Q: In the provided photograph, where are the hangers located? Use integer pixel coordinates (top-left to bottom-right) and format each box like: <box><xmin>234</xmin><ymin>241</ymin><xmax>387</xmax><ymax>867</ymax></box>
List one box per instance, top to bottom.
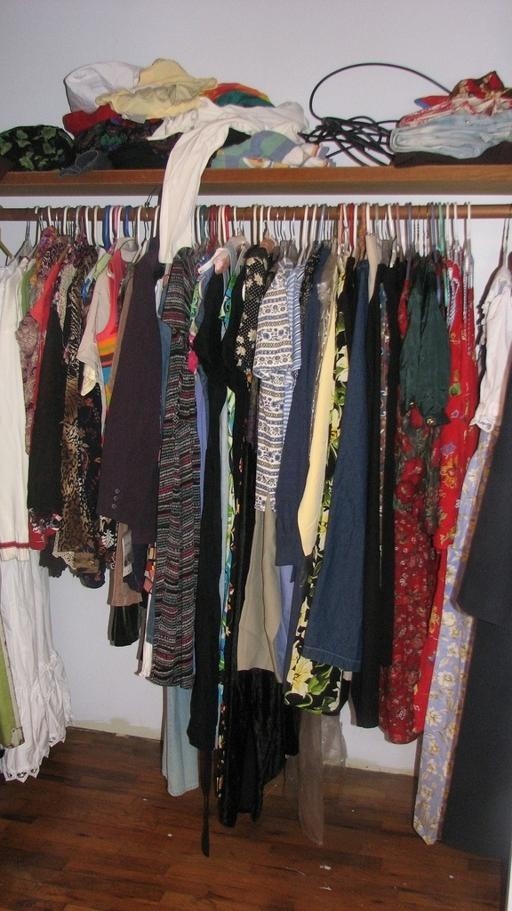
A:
<box><xmin>3</xmin><ymin>203</ymin><xmax>476</xmax><ymax>276</ymax></box>
<box><xmin>497</xmin><ymin>203</ymin><xmax>512</xmax><ymax>257</ymax></box>
